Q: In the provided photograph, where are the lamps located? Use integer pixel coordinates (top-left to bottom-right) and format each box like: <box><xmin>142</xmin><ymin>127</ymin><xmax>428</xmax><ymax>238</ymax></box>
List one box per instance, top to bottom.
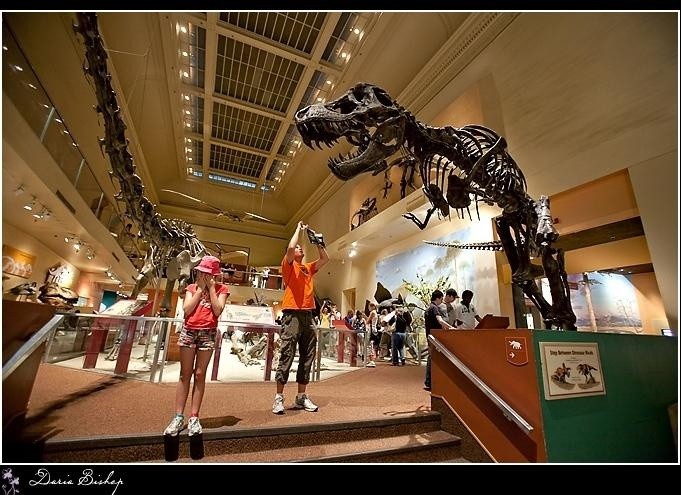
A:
<box><xmin>23</xmin><ymin>200</ymin><xmax>37</xmax><ymax>212</ymax></box>
<box><xmin>32</xmin><ymin>207</ymin><xmax>46</xmax><ymax>220</ymax></box>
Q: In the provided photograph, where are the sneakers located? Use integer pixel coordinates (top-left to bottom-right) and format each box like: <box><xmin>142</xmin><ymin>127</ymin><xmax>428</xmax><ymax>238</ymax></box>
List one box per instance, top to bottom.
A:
<box><xmin>366</xmin><ymin>362</ymin><xmax>376</xmax><ymax>368</ymax></box>
<box><xmin>294</xmin><ymin>395</ymin><xmax>319</xmax><ymax>412</ymax></box>
<box><xmin>271</xmin><ymin>397</ymin><xmax>285</xmax><ymax>414</ymax></box>
<box><xmin>163</xmin><ymin>416</ymin><xmax>186</xmax><ymax>437</ymax></box>
<box><xmin>187</xmin><ymin>416</ymin><xmax>203</xmax><ymax>437</ymax></box>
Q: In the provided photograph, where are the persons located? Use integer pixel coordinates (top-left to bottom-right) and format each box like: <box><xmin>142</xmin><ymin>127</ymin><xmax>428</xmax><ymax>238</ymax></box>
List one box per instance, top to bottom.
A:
<box><xmin>271</xmin><ymin>220</ymin><xmax>330</xmax><ymax>415</ymax></box>
<box><xmin>162</xmin><ymin>256</ymin><xmax>230</xmax><ymax>438</ymax></box>
<box><xmin>438</xmin><ymin>288</ymin><xmax>457</xmax><ymax>331</ymax></box>
<box><xmin>453</xmin><ymin>289</ymin><xmax>483</xmax><ymax>331</ymax></box>
<box><xmin>423</xmin><ymin>290</ymin><xmax>456</xmax><ymax>390</ymax></box>
<box><xmin>319</xmin><ymin>300</ymin><xmax>331</xmax><ymax>328</ymax></box>
<box><xmin>328</xmin><ymin>303</ymin><xmax>341</xmax><ymax>326</ymax></box>
<box><xmin>365</xmin><ymin>303</ymin><xmax>379</xmax><ymax>367</ymax></box>
<box><xmin>390</xmin><ymin>307</ymin><xmax>395</xmax><ymax>312</ymax></box>
<box><xmin>392</xmin><ymin>308</ymin><xmax>406</xmax><ymax>366</ymax></box>
<box><xmin>375</xmin><ymin>309</ymin><xmax>391</xmax><ymax>362</ymax></box>
<box><xmin>377</xmin><ymin>309</ymin><xmax>386</xmax><ymax>327</ymax></box>
<box><xmin>312</xmin><ymin>310</ymin><xmax>319</xmax><ymax>323</ymax></box>
<box><xmin>344</xmin><ymin>310</ymin><xmax>353</xmax><ymax>327</ymax></box>
<box><xmin>352</xmin><ymin>310</ymin><xmax>365</xmax><ymax>358</ymax></box>
<box><xmin>403</xmin><ymin>311</ymin><xmax>419</xmax><ymax>361</ymax></box>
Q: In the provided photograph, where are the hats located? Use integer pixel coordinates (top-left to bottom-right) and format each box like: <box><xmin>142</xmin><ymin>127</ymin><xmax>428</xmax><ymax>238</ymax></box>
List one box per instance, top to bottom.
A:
<box><xmin>194</xmin><ymin>254</ymin><xmax>225</xmax><ymax>278</ymax></box>
<box><xmin>447</xmin><ymin>288</ymin><xmax>460</xmax><ymax>298</ymax></box>
